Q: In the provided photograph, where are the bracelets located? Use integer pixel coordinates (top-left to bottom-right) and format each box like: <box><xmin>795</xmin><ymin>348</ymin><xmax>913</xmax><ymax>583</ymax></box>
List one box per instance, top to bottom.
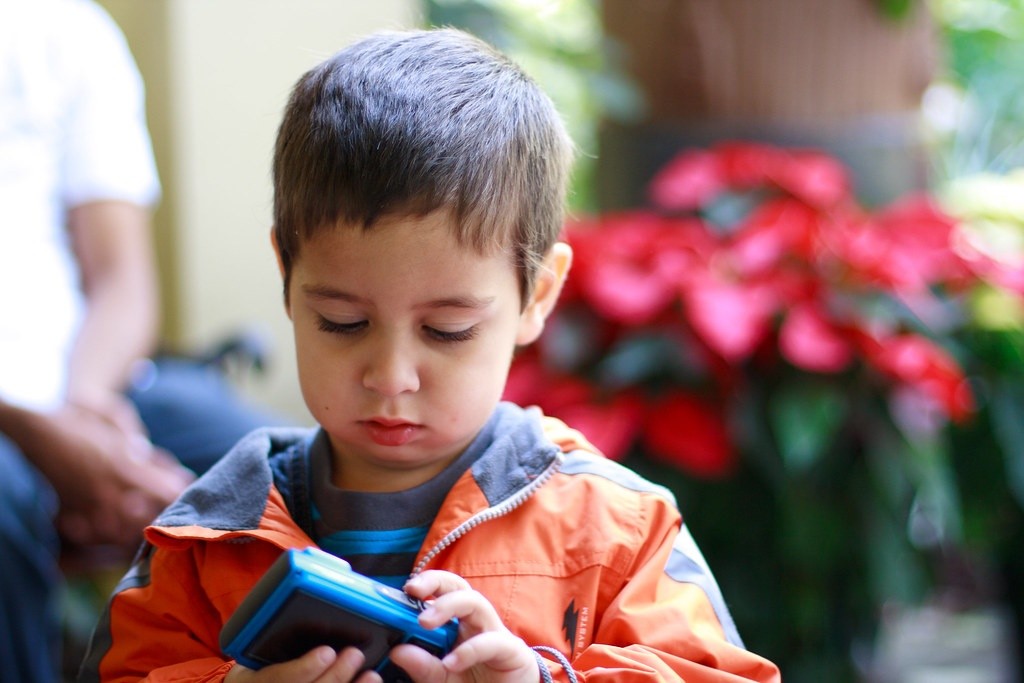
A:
<box><xmin>531</xmin><ymin>645</ymin><xmax>577</xmax><ymax>683</ymax></box>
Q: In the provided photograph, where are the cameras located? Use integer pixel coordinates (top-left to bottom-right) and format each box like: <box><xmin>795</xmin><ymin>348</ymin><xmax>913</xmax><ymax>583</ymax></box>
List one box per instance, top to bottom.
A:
<box><xmin>219</xmin><ymin>547</ymin><xmax>459</xmax><ymax>683</ymax></box>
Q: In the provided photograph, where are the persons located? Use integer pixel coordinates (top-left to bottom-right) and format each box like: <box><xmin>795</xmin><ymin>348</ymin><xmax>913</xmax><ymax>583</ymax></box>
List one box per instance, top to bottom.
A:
<box><xmin>0</xmin><ymin>0</ymin><xmax>276</xmax><ymax>683</ymax></box>
<box><xmin>77</xmin><ymin>24</ymin><xmax>782</xmax><ymax>682</ymax></box>
